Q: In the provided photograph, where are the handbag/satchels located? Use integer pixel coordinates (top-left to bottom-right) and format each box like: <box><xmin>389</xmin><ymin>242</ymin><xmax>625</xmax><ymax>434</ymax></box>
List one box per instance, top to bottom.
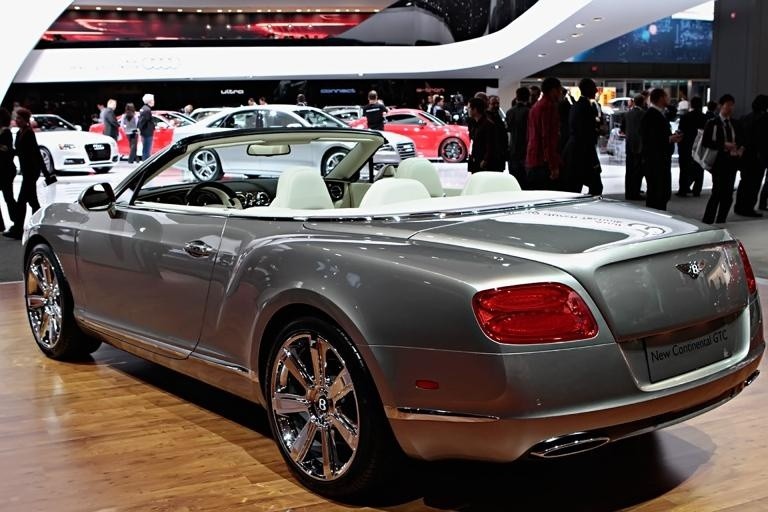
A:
<box><xmin>692</xmin><ymin>129</ymin><xmax>718</xmax><ymax>170</ymax></box>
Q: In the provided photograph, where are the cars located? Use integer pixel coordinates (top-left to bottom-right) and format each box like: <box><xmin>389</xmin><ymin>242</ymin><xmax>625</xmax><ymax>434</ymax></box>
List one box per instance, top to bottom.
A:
<box><xmin>39</xmin><ymin>0</ymin><xmax>397</xmax><ymax>44</ymax></box>
<box><xmin>601</xmin><ymin>97</ymin><xmax>634</xmax><ymax>120</ymax></box>
<box><xmin>169</xmin><ymin>103</ymin><xmax>417</xmax><ymax>179</ymax></box>
<box><xmin>349</xmin><ymin>108</ymin><xmax>472</xmax><ymax>164</ymax></box>
<box><xmin>88</xmin><ymin>111</ymin><xmax>198</xmax><ymax>162</ymax></box>
<box><xmin>9</xmin><ymin>113</ymin><xmax>120</xmax><ymax>177</ymax></box>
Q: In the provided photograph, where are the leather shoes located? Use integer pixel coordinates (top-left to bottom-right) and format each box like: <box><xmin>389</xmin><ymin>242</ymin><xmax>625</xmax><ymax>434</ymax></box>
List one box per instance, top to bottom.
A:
<box><xmin>1</xmin><ymin>228</ymin><xmax>22</xmax><ymax>239</ymax></box>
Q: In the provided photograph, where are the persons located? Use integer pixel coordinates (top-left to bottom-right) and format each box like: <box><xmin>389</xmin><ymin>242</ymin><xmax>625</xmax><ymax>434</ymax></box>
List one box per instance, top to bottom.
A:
<box><xmin>468</xmin><ymin>77</ymin><xmax>610</xmax><ymax>195</ymax></box>
<box><xmin>619</xmin><ymin>86</ymin><xmax>768</xmax><ymax>225</ymax></box>
<box><xmin>363</xmin><ymin>89</ymin><xmax>386</xmax><ymax>130</ymax></box>
<box><xmin>136</xmin><ymin>93</ymin><xmax>155</xmax><ymax>162</ymax></box>
<box><xmin>296</xmin><ymin>94</ymin><xmax>308</xmax><ymax>106</ymax></box>
<box><xmin>419</xmin><ymin>94</ymin><xmax>470</xmax><ymax>126</ymax></box>
<box><xmin>258</xmin><ymin>97</ymin><xmax>268</xmax><ymax>106</ymax></box>
<box><xmin>248</xmin><ymin>98</ymin><xmax>257</xmax><ymax>106</ymax></box>
<box><xmin>102</xmin><ymin>99</ymin><xmax>121</xmax><ymax>143</ymax></box>
<box><xmin>121</xmin><ymin>102</ymin><xmax>139</xmax><ymax>163</ymax></box>
<box><xmin>90</xmin><ymin>104</ymin><xmax>106</xmax><ymax>123</ymax></box>
<box><xmin>183</xmin><ymin>104</ymin><xmax>193</xmax><ymax>116</ymax></box>
<box><xmin>0</xmin><ymin>105</ymin><xmax>17</xmax><ymax>235</ymax></box>
<box><xmin>3</xmin><ymin>107</ymin><xmax>54</xmax><ymax>240</ymax></box>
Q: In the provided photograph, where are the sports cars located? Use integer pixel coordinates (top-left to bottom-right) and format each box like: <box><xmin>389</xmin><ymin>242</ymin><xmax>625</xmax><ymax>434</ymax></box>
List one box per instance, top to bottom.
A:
<box><xmin>20</xmin><ymin>127</ymin><xmax>766</xmax><ymax>498</ymax></box>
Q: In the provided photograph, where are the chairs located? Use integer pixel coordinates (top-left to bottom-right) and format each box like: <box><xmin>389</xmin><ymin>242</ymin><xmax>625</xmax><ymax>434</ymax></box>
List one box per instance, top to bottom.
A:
<box><xmin>392</xmin><ymin>157</ymin><xmax>444</xmax><ymax>197</ymax></box>
<box><xmin>268</xmin><ymin>168</ymin><xmax>335</xmax><ymax>209</ymax></box>
<box><xmin>462</xmin><ymin>171</ymin><xmax>522</xmax><ymax>197</ymax></box>
<box><xmin>356</xmin><ymin>177</ymin><xmax>432</xmax><ymax>209</ymax></box>
